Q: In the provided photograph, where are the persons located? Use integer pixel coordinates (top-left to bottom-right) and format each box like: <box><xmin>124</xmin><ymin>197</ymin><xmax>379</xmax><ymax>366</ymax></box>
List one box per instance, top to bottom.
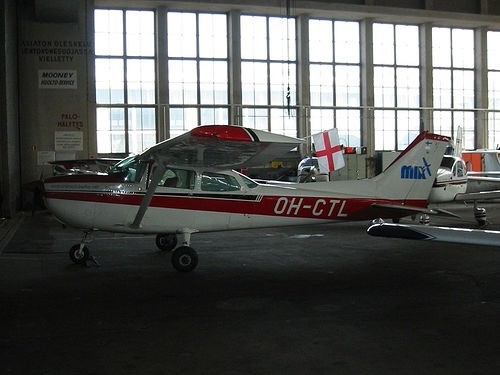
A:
<box><xmin>465</xmin><ymin>160</ymin><xmax>472</xmax><ymax>172</ymax></box>
<box><xmin>299</xmin><ymin>167</ymin><xmax>318</xmax><ymax>184</ymax></box>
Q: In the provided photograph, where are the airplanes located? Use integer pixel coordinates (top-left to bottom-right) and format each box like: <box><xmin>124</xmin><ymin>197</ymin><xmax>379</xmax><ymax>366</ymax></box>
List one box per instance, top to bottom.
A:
<box><xmin>24</xmin><ymin>124</ymin><xmax>500</xmax><ymax>272</ymax></box>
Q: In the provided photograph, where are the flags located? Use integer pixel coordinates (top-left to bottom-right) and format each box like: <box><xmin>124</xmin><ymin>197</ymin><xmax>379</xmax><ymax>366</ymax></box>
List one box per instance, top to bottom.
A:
<box><xmin>311</xmin><ymin>127</ymin><xmax>345</xmax><ymax>174</ymax></box>
